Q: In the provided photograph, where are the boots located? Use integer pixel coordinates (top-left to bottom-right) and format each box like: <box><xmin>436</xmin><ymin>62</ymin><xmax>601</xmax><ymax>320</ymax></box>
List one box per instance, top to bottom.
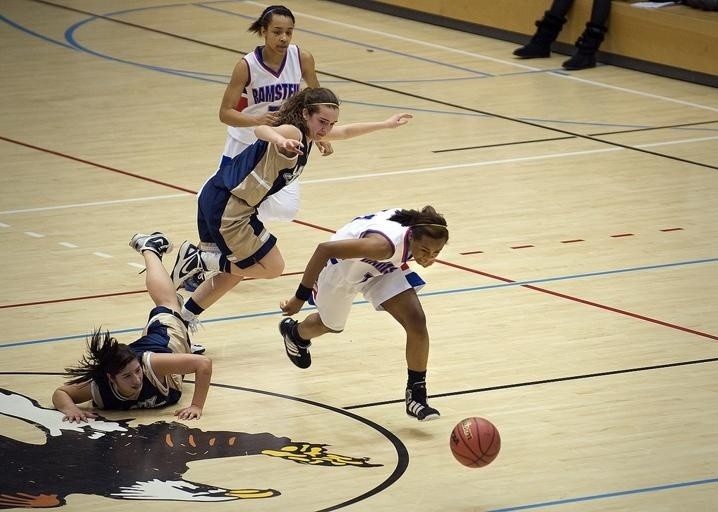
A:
<box><xmin>511</xmin><ymin>9</ymin><xmax>569</xmax><ymax>60</ymax></box>
<box><xmin>561</xmin><ymin>22</ymin><xmax>608</xmax><ymax>71</ymax></box>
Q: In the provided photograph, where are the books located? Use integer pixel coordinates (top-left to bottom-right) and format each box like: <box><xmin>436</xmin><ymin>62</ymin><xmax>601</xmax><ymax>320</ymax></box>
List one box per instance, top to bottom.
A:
<box><xmin>629</xmin><ymin>2</ymin><xmax>676</xmax><ymax>10</ymax></box>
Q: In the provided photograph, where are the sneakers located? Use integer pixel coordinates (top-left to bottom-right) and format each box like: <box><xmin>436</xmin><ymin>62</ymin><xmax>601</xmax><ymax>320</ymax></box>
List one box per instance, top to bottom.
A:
<box><xmin>128</xmin><ymin>230</ymin><xmax>175</xmax><ymax>259</ymax></box>
<box><xmin>168</xmin><ymin>241</ymin><xmax>204</xmax><ymax>311</ymax></box>
<box><xmin>278</xmin><ymin>317</ymin><xmax>313</xmax><ymax>369</ymax></box>
<box><xmin>188</xmin><ymin>341</ymin><xmax>207</xmax><ymax>356</ymax></box>
<box><xmin>403</xmin><ymin>379</ymin><xmax>441</xmax><ymax>422</ymax></box>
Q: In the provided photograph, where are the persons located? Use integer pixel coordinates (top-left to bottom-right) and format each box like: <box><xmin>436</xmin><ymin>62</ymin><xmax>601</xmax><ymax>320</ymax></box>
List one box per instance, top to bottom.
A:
<box><xmin>511</xmin><ymin>0</ymin><xmax>614</xmax><ymax>71</ymax></box>
<box><xmin>182</xmin><ymin>7</ymin><xmax>333</xmax><ymax>295</ymax></box>
<box><xmin>170</xmin><ymin>88</ymin><xmax>413</xmax><ymax>353</ymax></box>
<box><xmin>277</xmin><ymin>205</ymin><xmax>450</xmax><ymax>420</ymax></box>
<box><xmin>52</xmin><ymin>232</ymin><xmax>214</xmax><ymax>425</ymax></box>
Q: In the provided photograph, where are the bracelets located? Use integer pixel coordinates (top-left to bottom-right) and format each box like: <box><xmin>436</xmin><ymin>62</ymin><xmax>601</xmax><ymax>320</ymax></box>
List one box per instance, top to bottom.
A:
<box><xmin>295</xmin><ymin>283</ymin><xmax>312</xmax><ymax>301</ymax></box>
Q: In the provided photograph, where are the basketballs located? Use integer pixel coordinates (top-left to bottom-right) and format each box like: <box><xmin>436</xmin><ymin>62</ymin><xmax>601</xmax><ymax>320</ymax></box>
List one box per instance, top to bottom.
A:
<box><xmin>450</xmin><ymin>417</ymin><xmax>500</xmax><ymax>467</ymax></box>
